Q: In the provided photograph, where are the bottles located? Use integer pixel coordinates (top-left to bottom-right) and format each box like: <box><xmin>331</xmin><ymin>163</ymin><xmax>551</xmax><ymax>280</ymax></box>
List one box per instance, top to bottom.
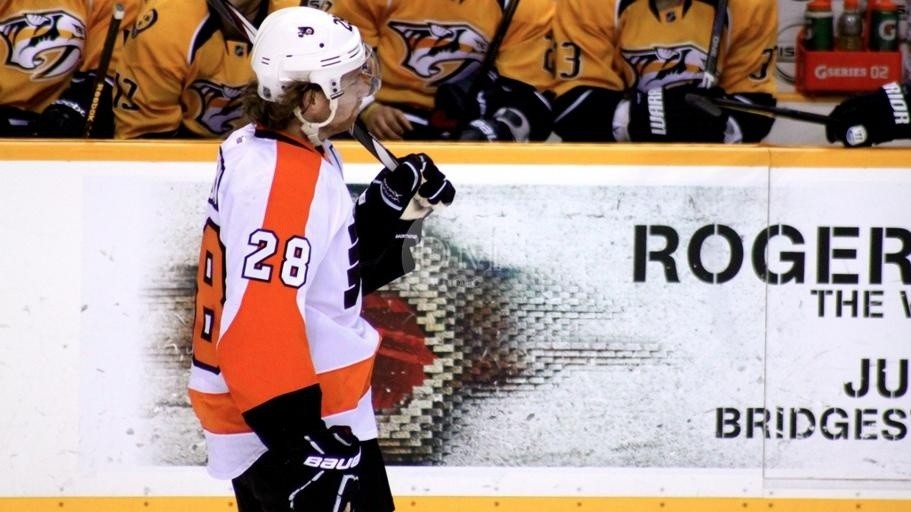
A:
<box><xmin>804</xmin><ymin>1</ymin><xmax>899</xmax><ymax>51</ymax></box>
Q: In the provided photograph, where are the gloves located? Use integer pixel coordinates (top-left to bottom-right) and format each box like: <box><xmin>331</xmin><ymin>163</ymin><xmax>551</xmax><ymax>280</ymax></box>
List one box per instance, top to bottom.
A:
<box><xmin>822</xmin><ymin>79</ymin><xmax>911</xmax><ymax>147</ymax></box>
<box><xmin>459</xmin><ymin>85</ymin><xmax>552</xmax><ymax>142</ymax></box>
<box><xmin>629</xmin><ymin>88</ymin><xmax>729</xmax><ymax>142</ymax></box>
<box><xmin>724</xmin><ymin>92</ymin><xmax>778</xmax><ymax>141</ymax></box>
<box><xmin>354</xmin><ymin>152</ymin><xmax>455</xmax><ymax>247</ymax></box>
<box><xmin>272</xmin><ymin>439</ymin><xmax>363</xmax><ymax>512</ymax></box>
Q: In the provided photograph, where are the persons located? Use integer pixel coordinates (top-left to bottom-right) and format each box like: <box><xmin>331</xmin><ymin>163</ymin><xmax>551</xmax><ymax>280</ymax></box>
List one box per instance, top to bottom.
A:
<box><xmin>3</xmin><ymin>1</ymin><xmax>780</xmax><ymax>143</ymax></box>
<box><xmin>187</xmin><ymin>5</ymin><xmax>458</xmax><ymax>511</ymax></box>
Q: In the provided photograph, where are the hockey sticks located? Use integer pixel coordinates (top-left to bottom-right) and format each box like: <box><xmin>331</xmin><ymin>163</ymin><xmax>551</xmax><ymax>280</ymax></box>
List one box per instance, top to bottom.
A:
<box><xmin>685</xmin><ymin>91</ymin><xmax>828</xmax><ymax>126</ymax></box>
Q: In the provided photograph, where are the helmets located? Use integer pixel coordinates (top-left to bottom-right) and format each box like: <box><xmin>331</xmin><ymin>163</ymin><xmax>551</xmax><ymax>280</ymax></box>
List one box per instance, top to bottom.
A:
<box><xmin>251</xmin><ymin>5</ymin><xmax>371</xmax><ymax>105</ymax></box>
